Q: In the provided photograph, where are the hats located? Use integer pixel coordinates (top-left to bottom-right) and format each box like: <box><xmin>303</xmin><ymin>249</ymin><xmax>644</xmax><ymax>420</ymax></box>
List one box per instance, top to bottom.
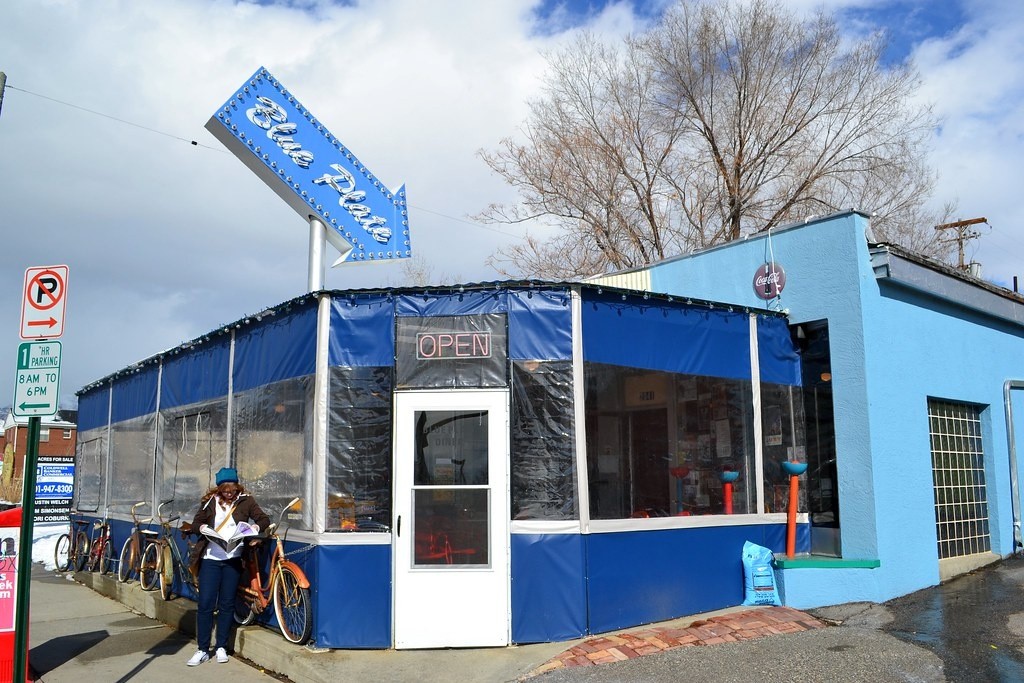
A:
<box><xmin>215</xmin><ymin>466</ymin><xmax>239</xmax><ymax>486</ymax></box>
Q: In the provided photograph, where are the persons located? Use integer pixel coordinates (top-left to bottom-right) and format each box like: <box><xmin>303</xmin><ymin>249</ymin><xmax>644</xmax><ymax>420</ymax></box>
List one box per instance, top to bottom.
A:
<box><xmin>187</xmin><ymin>467</ymin><xmax>270</xmax><ymax>666</ymax></box>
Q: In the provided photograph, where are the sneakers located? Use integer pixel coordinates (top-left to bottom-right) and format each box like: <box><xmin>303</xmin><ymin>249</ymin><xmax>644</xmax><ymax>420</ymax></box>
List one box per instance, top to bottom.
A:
<box><xmin>216</xmin><ymin>647</ymin><xmax>229</xmax><ymax>663</ymax></box>
<box><xmin>187</xmin><ymin>650</ymin><xmax>209</xmax><ymax>665</ymax></box>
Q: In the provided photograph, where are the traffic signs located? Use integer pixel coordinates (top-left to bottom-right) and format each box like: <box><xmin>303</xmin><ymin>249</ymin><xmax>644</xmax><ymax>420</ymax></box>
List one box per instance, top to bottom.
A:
<box><xmin>12</xmin><ymin>341</ymin><xmax>62</xmax><ymax>416</ymax></box>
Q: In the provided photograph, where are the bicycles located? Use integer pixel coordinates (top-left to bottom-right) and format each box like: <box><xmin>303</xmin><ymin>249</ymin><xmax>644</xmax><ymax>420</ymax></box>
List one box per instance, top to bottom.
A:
<box><xmin>234</xmin><ymin>496</ymin><xmax>313</xmax><ymax>645</ymax></box>
<box><xmin>157</xmin><ymin>499</ymin><xmax>199</xmax><ymax>601</ymax></box>
<box><xmin>117</xmin><ymin>501</ymin><xmax>160</xmax><ymax>591</ymax></box>
<box><xmin>86</xmin><ymin>503</ymin><xmax>113</xmax><ymax>575</ymax></box>
<box><xmin>54</xmin><ymin>511</ymin><xmax>89</xmax><ymax>573</ymax></box>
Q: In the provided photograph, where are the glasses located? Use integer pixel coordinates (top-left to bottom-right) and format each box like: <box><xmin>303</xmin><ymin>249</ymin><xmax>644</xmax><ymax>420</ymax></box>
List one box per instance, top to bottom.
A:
<box><xmin>221</xmin><ymin>490</ymin><xmax>238</xmax><ymax>495</ymax></box>
